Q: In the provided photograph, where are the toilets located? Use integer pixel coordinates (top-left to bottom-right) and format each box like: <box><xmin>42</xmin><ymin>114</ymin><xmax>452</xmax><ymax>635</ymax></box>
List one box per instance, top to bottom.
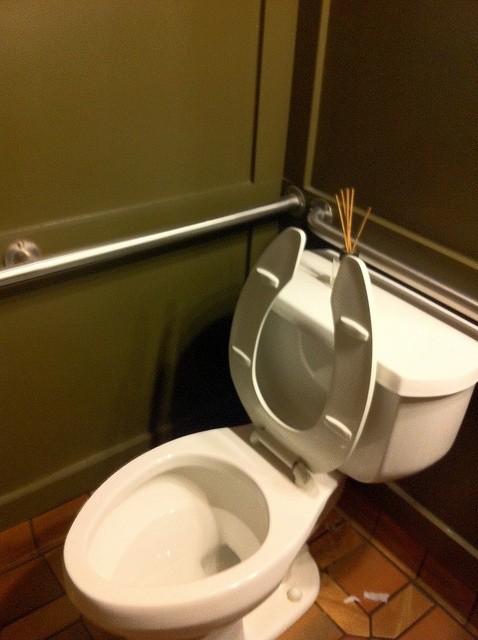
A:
<box><xmin>59</xmin><ymin>227</ymin><xmax>478</xmax><ymax>640</ymax></box>
